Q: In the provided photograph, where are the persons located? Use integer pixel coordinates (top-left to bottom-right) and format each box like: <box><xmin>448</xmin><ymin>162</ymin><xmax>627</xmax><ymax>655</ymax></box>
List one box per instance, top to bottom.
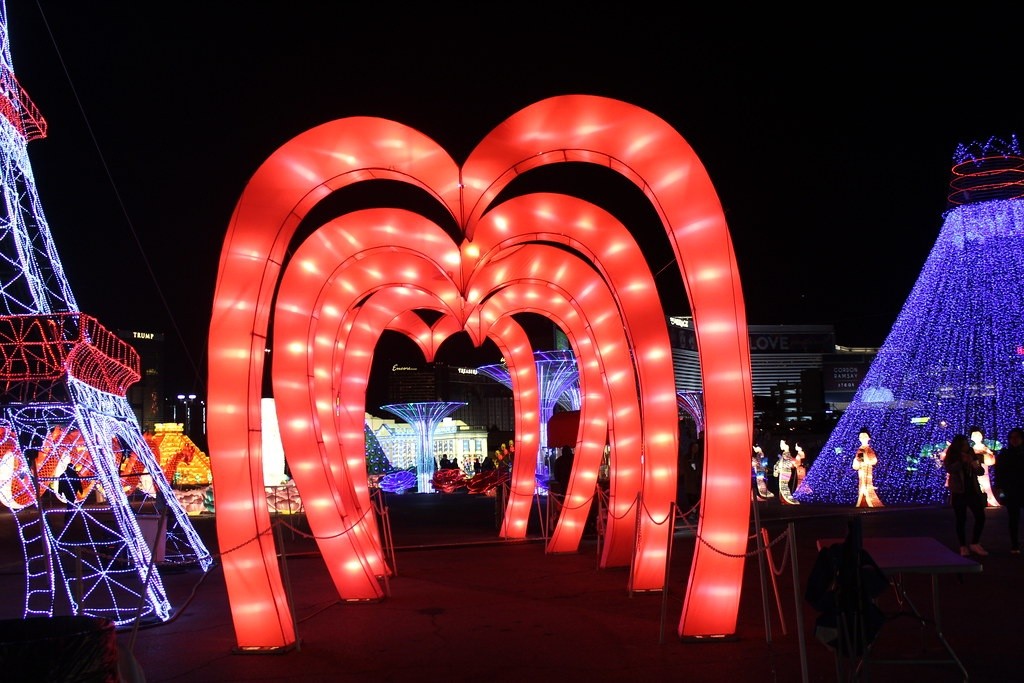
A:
<box><xmin>433</xmin><ymin>426</ymin><xmax>705</xmax><ymax>499</ymax></box>
<box><xmin>851</xmin><ymin>426</ymin><xmax>884</xmax><ymax>508</ymax></box>
<box><xmin>965</xmin><ymin>426</ymin><xmax>1001</xmax><ymax>507</ymax></box>
<box><xmin>994</xmin><ymin>427</ymin><xmax>1023</xmax><ymax>556</ymax></box>
<box><xmin>943</xmin><ymin>433</ymin><xmax>989</xmax><ymax>558</ymax></box>
<box><xmin>773</xmin><ymin>437</ymin><xmax>800</xmax><ymax>507</ymax></box>
<box><xmin>792</xmin><ymin>441</ymin><xmax>806</xmax><ymax>496</ymax></box>
<box><xmin>751</xmin><ymin>442</ymin><xmax>775</xmax><ymax>498</ymax></box>
<box><xmin>58</xmin><ymin>463</ymin><xmax>83</xmax><ymax>527</ymax></box>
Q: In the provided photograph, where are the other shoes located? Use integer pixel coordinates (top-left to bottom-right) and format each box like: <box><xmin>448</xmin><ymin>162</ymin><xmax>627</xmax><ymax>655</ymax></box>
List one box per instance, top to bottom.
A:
<box><xmin>961</xmin><ymin>544</ymin><xmax>989</xmax><ymax>557</ymax></box>
